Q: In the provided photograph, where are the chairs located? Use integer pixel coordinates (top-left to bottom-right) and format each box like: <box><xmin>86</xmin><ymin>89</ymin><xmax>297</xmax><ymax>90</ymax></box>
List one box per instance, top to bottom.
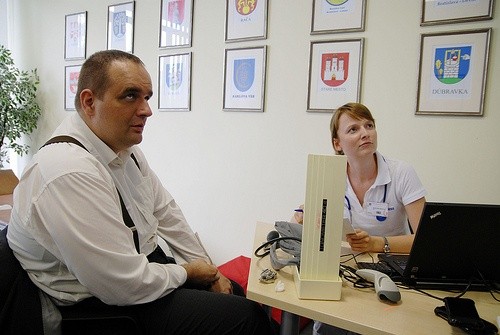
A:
<box><xmin>0</xmin><ymin>226</ymin><xmax>141</xmax><ymax>335</ymax></box>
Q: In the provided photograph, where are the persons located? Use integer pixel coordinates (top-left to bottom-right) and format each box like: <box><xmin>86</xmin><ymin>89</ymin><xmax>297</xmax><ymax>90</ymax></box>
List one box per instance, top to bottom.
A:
<box><xmin>6</xmin><ymin>49</ymin><xmax>277</xmax><ymax>335</ymax></box>
<box><xmin>290</xmin><ymin>103</ymin><xmax>427</xmax><ymax>253</ymax></box>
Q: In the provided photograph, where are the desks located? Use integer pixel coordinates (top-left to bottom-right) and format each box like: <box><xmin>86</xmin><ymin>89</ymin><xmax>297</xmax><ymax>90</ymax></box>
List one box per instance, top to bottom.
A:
<box><xmin>246</xmin><ymin>221</ymin><xmax>500</xmax><ymax>335</ymax></box>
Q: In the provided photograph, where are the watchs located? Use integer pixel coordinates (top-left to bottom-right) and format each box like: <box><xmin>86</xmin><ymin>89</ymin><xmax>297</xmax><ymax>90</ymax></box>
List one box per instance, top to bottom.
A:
<box><xmin>383</xmin><ymin>235</ymin><xmax>390</xmax><ymax>253</ymax></box>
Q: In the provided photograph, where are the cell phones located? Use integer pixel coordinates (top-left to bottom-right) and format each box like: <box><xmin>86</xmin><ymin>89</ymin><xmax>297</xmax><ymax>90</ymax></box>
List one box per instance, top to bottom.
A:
<box><xmin>444</xmin><ymin>295</ymin><xmax>483</xmax><ymax>326</ymax></box>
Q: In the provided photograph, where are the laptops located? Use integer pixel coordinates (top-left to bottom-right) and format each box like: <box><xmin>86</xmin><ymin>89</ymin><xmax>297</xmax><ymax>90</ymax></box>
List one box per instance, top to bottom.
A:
<box><xmin>377</xmin><ymin>201</ymin><xmax>500</xmax><ymax>291</ymax></box>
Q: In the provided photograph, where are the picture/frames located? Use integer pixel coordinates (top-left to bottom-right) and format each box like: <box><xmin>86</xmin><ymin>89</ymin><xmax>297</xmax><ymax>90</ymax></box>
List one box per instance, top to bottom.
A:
<box><xmin>158</xmin><ymin>0</ymin><xmax>194</xmax><ymax>50</ymax></box>
<box><xmin>224</xmin><ymin>0</ymin><xmax>269</xmax><ymax>44</ymax></box>
<box><xmin>310</xmin><ymin>0</ymin><xmax>367</xmax><ymax>35</ymax></box>
<box><xmin>419</xmin><ymin>0</ymin><xmax>494</xmax><ymax>26</ymax></box>
<box><xmin>106</xmin><ymin>1</ymin><xmax>137</xmax><ymax>55</ymax></box>
<box><xmin>63</xmin><ymin>10</ymin><xmax>88</xmax><ymax>61</ymax></box>
<box><xmin>414</xmin><ymin>27</ymin><xmax>492</xmax><ymax>116</ymax></box>
<box><xmin>305</xmin><ymin>37</ymin><xmax>365</xmax><ymax>113</ymax></box>
<box><xmin>222</xmin><ymin>45</ymin><xmax>266</xmax><ymax>112</ymax></box>
<box><xmin>157</xmin><ymin>52</ymin><xmax>192</xmax><ymax>111</ymax></box>
<box><xmin>64</xmin><ymin>64</ymin><xmax>82</xmax><ymax>111</ymax></box>
